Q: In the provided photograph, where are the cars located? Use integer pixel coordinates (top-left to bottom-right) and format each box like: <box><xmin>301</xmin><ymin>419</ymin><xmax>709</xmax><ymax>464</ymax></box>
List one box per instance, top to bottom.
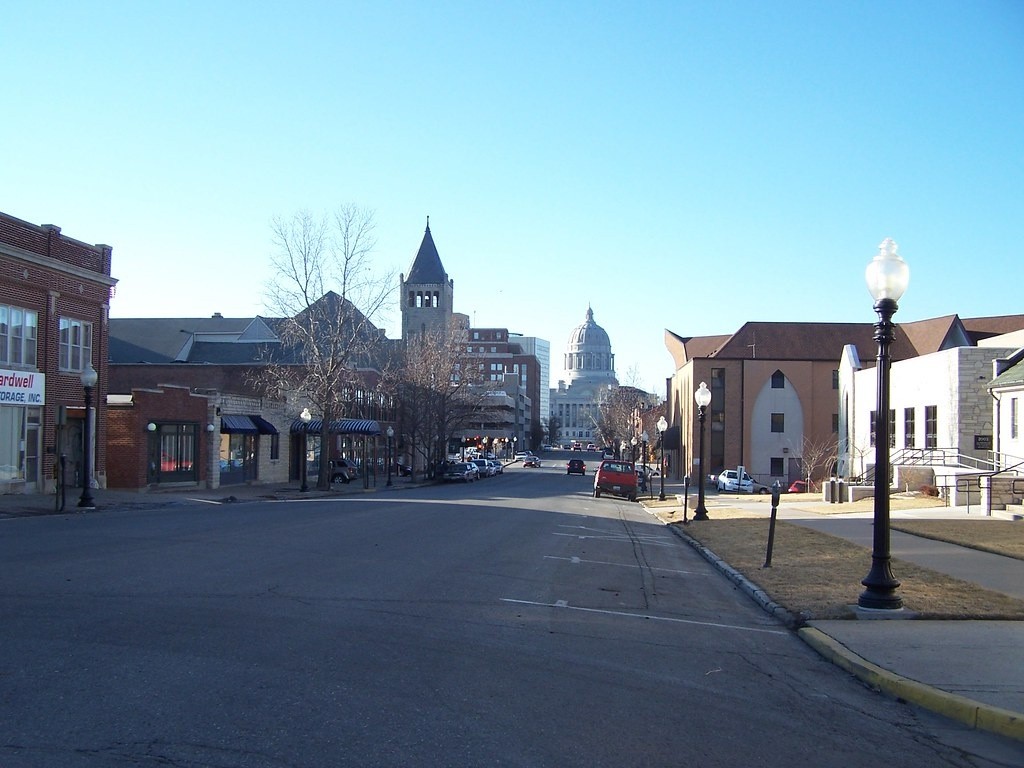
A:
<box><xmin>563</xmin><ymin>443</ymin><xmax>597</xmax><ymax>452</ymax></box>
<box><xmin>515</xmin><ymin>450</ymin><xmax>541</xmax><ymax>468</ymax></box>
<box><xmin>441</xmin><ymin>458</ymin><xmax>489</xmax><ymax>484</ymax></box>
<box><xmin>487</xmin><ymin>460</ymin><xmax>503</xmax><ymax>477</ymax></box>
<box><xmin>567</xmin><ymin>460</ymin><xmax>586</xmax><ymax>475</ymax></box>
<box><xmin>635</xmin><ymin>465</ymin><xmax>661</xmax><ymax>477</ymax></box>
<box><xmin>717</xmin><ymin>470</ymin><xmax>754</xmax><ymax>494</ymax></box>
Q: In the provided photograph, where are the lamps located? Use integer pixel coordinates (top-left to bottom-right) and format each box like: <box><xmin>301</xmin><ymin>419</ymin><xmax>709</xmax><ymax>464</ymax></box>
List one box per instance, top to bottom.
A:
<box><xmin>147</xmin><ymin>422</ymin><xmax>157</xmax><ymax>432</ymax></box>
<box><xmin>206</xmin><ymin>423</ymin><xmax>215</xmax><ymax>432</ymax></box>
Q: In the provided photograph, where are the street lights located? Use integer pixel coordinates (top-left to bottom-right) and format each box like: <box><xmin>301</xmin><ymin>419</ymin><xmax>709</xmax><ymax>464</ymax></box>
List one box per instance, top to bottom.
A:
<box><xmin>857</xmin><ymin>239</ymin><xmax>907</xmax><ymax>609</ymax></box>
<box><xmin>78</xmin><ymin>362</ymin><xmax>96</xmax><ymax>507</ymax></box>
<box><xmin>693</xmin><ymin>382</ymin><xmax>711</xmax><ymax>520</ymax></box>
<box><xmin>300</xmin><ymin>408</ymin><xmax>311</xmax><ymax>492</ymax></box>
<box><xmin>656</xmin><ymin>416</ymin><xmax>667</xmax><ymax>501</ymax></box>
<box><xmin>385</xmin><ymin>426</ymin><xmax>395</xmax><ymax>486</ymax></box>
<box><xmin>641</xmin><ymin>430</ymin><xmax>648</xmax><ymax>493</ymax></box>
<box><xmin>482</xmin><ymin>437</ymin><xmax>518</xmax><ymax>464</ymax></box>
<box><xmin>631</xmin><ymin>437</ymin><xmax>638</xmax><ymax>464</ymax></box>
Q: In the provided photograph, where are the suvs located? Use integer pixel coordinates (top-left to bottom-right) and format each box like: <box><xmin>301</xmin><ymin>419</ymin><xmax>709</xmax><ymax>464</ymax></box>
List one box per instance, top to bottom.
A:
<box><xmin>329</xmin><ymin>457</ymin><xmax>360</xmax><ymax>484</ymax></box>
<box><xmin>593</xmin><ymin>460</ymin><xmax>637</xmax><ymax>501</ymax></box>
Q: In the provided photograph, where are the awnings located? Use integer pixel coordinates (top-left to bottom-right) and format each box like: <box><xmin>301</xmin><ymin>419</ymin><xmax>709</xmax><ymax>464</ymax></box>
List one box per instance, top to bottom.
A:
<box><xmin>220</xmin><ymin>416</ymin><xmax>258</xmax><ymax>435</ymax></box>
<box><xmin>249</xmin><ymin>416</ymin><xmax>277</xmax><ymax>435</ymax></box>
<box><xmin>291</xmin><ymin>420</ymin><xmax>381</xmax><ymax>489</ymax></box>
<box><xmin>661</xmin><ymin>426</ymin><xmax>680</xmax><ymax>450</ymax></box>
